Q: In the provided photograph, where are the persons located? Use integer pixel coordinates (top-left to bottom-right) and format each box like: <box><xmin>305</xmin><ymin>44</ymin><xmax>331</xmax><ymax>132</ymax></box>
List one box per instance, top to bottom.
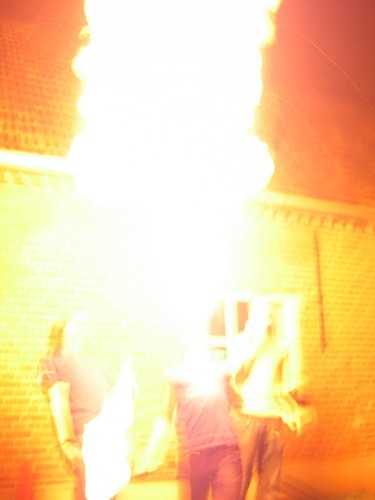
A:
<box><xmin>41</xmin><ymin>320</ymin><xmax>116</xmax><ymax>500</ymax></box>
<box><xmin>165</xmin><ymin>331</ymin><xmax>246</xmax><ymax>500</ymax></box>
